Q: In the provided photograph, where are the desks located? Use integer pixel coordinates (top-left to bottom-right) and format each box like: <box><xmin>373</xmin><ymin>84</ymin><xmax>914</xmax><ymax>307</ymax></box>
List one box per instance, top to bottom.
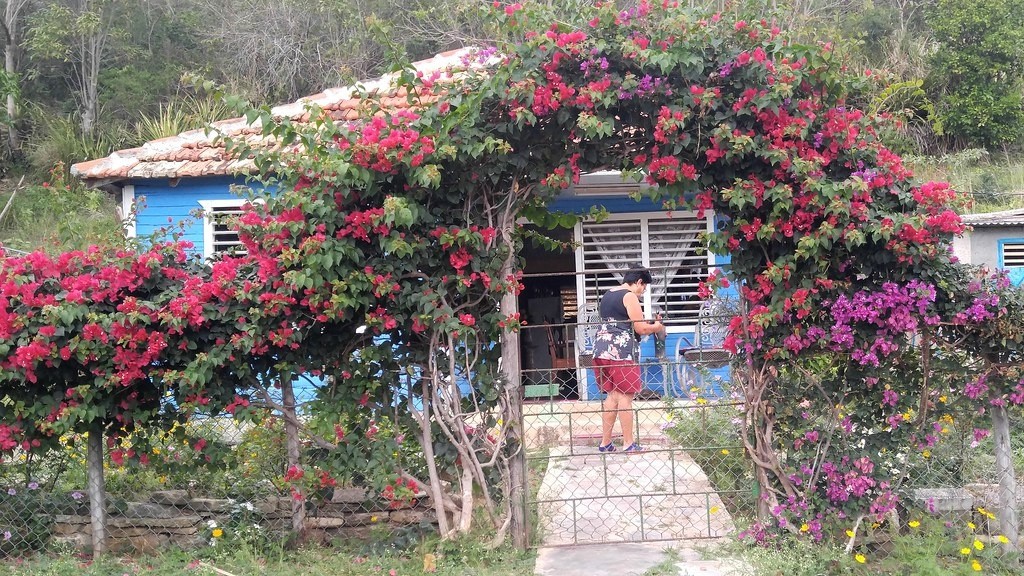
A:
<box><xmin>645</xmin><ymin>358</ymin><xmax>680</xmax><ymax>398</ymax></box>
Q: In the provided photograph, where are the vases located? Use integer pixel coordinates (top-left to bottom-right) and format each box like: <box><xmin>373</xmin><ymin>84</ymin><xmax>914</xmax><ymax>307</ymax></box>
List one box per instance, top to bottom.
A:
<box><xmin>654</xmin><ymin>333</ymin><xmax>665</xmax><ymax>358</ymax></box>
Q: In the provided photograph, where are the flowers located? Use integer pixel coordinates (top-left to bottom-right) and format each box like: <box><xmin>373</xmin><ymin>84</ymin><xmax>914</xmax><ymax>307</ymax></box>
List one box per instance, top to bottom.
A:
<box><xmin>654</xmin><ymin>312</ymin><xmax>665</xmax><ymax>323</ymax></box>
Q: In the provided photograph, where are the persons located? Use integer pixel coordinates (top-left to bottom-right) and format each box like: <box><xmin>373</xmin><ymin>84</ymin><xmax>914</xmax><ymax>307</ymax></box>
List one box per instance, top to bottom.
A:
<box><xmin>592</xmin><ymin>265</ymin><xmax>664</xmax><ymax>455</ymax></box>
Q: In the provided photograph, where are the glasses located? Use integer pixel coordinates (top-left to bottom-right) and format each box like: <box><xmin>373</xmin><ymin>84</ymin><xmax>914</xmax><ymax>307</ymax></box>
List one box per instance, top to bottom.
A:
<box><xmin>635</xmin><ymin>281</ymin><xmax>647</xmax><ymax>293</ymax></box>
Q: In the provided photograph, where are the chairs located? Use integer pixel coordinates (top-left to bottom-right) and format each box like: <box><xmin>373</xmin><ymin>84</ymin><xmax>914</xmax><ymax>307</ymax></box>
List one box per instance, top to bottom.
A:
<box><xmin>675</xmin><ymin>298</ymin><xmax>739</xmax><ymax>399</ymax></box>
<box><xmin>545</xmin><ymin>320</ymin><xmax>575</xmax><ymax>384</ymax></box>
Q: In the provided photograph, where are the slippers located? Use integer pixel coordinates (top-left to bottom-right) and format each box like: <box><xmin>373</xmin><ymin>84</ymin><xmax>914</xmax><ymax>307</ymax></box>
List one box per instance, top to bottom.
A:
<box><xmin>599</xmin><ymin>442</ymin><xmax>620</xmax><ymax>452</ymax></box>
<box><xmin>624</xmin><ymin>443</ymin><xmax>648</xmax><ymax>452</ymax></box>
